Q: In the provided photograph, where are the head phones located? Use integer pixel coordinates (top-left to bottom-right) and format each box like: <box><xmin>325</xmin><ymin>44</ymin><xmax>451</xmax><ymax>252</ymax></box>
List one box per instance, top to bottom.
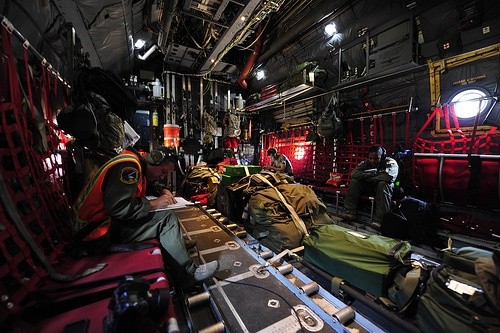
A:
<box><xmin>146</xmin><ymin>126</ymin><xmax>168</xmax><ymax>166</ymax></box>
<box><xmin>366</xmin><ymin>145</ymin><xmax>387</xmax><ymax>161</ymax></box>
<box><xmin>268</xmin><ymin>149</ymin><xmax>277</xmax><ymax>158</ymax></box>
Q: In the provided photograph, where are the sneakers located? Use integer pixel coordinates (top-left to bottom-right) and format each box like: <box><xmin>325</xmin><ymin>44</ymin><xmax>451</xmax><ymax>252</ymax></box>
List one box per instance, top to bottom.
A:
<box><xmin>184</xmin><ymin>239</ymin><xmax>197</xmax><ymax>250</ymax></box>
<box><xmin>181</xmin><ymin>260</ymin><xmax>219</xmax><ymax>293</ymax></box>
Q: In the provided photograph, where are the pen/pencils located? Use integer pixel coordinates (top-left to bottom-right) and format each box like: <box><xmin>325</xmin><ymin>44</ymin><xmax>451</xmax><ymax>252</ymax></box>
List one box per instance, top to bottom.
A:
<box><xmin>156</xmin><ymin>190</ymin><xmax>175</xmax><ymax>206</ymax></box>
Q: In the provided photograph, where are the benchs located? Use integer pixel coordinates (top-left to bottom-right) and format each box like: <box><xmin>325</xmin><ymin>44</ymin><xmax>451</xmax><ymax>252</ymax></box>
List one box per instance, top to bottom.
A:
<box><xmin>316</xmin><ymin>184</ymin><xmax>375</xmax><ymax>221</ymax></box>
<box><xmin>10</xmin><ymin>237</ymin><xmax>181</xmax><ymax>333</ymax></box>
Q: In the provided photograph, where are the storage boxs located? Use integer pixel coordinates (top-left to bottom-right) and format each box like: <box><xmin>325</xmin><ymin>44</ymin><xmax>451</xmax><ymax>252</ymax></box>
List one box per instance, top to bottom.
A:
<box><xmin>217</xmin><ymin>164</ymin><xmax>262</xmax><ymax>218</ymax></box>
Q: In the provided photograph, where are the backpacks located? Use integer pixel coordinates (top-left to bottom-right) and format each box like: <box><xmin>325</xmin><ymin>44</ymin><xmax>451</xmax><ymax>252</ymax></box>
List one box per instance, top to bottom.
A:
<box><xmin>85</xmin><ymin>67</ymin><xmax>139</xmax><ymax>122</ymax></box>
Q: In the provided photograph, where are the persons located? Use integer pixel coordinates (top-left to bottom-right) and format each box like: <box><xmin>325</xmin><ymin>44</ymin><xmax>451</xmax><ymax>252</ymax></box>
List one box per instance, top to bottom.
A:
<box><xmin>342</xmin><ymin>146</ymin><xmax>399</xmax><ymax>226</ymax></box>
<box><xmin>73</xmin><ymin>148</ymin><xmax>219</xmax><ymax>292</ymax></box>
<box><xmin>262</xmin><ymin>148</ymin><xmax>293</xmax><ymax>174</ymax></box>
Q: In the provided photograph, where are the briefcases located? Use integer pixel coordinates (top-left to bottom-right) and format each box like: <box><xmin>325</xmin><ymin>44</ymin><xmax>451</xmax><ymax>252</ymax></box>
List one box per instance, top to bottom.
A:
<box><xmin>225</xmin><ymin>175</ymin><xmax>271</xmax><ymax>223</ymax></box>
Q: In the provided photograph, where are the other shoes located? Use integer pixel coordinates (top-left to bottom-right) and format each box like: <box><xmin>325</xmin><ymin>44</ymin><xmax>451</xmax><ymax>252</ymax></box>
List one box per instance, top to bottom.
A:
<box><xmin>341</xmin><ymin>213</ymin><xmax>356</xmax><ymax>220</ymax></box>
<box><xmin>370</xmin><ymin>220</ymin><xmax>382</xmax><ymax>230</ymax></box>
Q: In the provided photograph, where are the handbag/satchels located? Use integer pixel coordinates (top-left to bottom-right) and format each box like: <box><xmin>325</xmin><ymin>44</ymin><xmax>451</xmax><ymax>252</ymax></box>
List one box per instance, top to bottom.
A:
<box><xmin>316</xmin><ymin>95</ymin><xmax>343</xmax><ymax>138</ymax></box>
<box><xmin>247</xmin><ymin>183</ymin><xmax>500</xmax><ymax>333</ymax></box>
